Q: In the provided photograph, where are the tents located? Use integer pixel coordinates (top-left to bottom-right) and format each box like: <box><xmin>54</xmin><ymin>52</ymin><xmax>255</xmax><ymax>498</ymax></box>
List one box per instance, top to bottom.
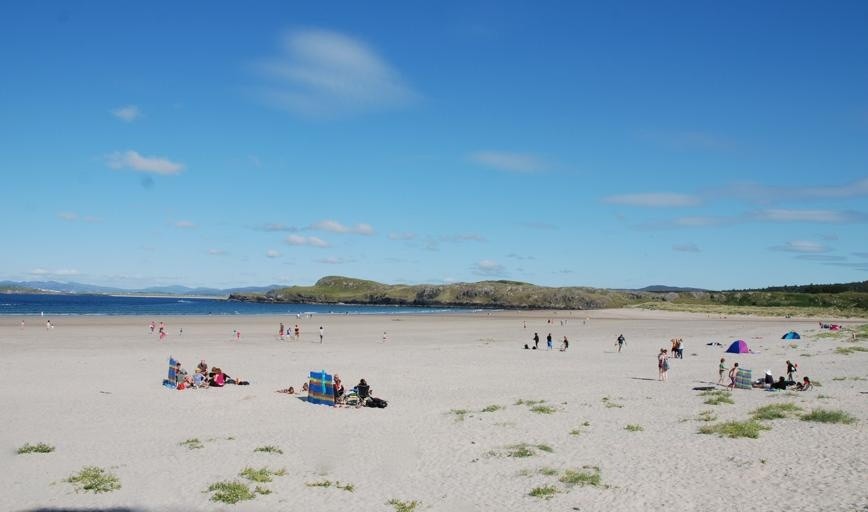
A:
<box><xmin>781</xmin><ymin>331</ymin><xmax>800</xmax><ymax>339</ymax></box>
<box><xmin>726</xmin><ymin>340</ymin><xmax>748</xmax><ymax>353</ymax></box>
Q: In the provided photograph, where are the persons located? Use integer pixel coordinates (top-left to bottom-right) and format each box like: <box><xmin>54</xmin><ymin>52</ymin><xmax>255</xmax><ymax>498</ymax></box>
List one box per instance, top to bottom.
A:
<box><xmin>20</xmin><ymin>320</ymin><xmax>24</xmax><ymax>330</ymax></box>
<box><xmin>46</xmin><ymin>320</ymin><xmax>50</xmax><ymax>331</ymax></box>
<box><xmin>151</xmin><ymin>321</ymin><xmax>182</xmax><ymax>343</ymax></box>
<box><xmin>278</xmin><ymin>323</ymin><xmax>299</xmax><ymax>340</ymax></box>
<box><xmin>232</xmin><ymin>327</ymin><xmax>240</xmax><ymax>342</ymax></box>
<box><xmin>319</xmin><ymin>327</ymin><xmax>323</xmax><ymax>343</ymax></box>
<box><xmin>533</xmin><ymin>333</ymin><xmax>539</xmax><ymax>346</ymax></box>
<box><xmin>547</xmin><ymin>333</ymin><xmax>551</xmax><ymax>347</ymax></box>
<box><xmin>615</xmin><ymin>334</ymin><xmax>626</xmax><ymax>352</ymax></box>
<box><xmin>564</xmin><ymin>336</ymin><xmax>568</xmax><ymax>348</ymax></box>
<box><xmin>658</xmin><ymin>338</ymin><xmax>683</xmax><ymax>381</ymax></box>
<box><xmin>719</xmin><ymin>358</ymin><xmax>813</xmax><ymax>392</ymax></box>
<box><xmin>176</xmin><ymin>360</ymin><xmax>240</xmax><ymax>391</ymax></box>
<box><xmin>333</xmin><ymin>374</ymin><xmax>370</xmax><ymax>399</ymax></box>
<box><xmin>303</xmin><ymin>383</ymin><xmax>308</xmax><ymax>391</ymax></box>
<box><xmin>288</xmin><ymin>387</ymin><xmax>294</xmax><ymax>394</ymax></box>
